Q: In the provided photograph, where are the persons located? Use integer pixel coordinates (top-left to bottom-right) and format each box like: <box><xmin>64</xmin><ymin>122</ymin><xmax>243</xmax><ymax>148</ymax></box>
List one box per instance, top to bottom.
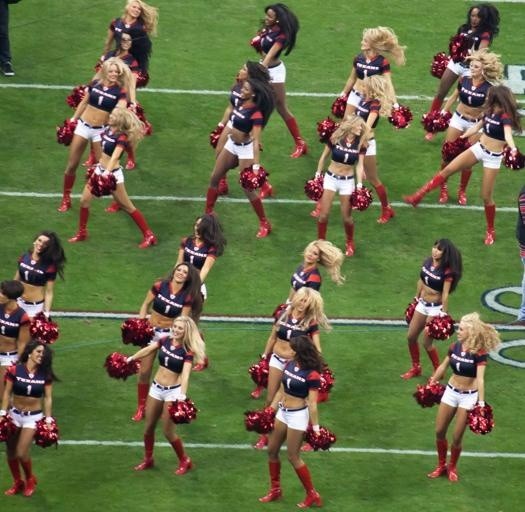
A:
<box><xmin>57</xmin><ymin>0</ymin><xmax>159</xmax><ymax>248</ymax></box>
<box><xmin>1</xmin><ymin>1</ymin><xmax>17</xmax><ymax>76</ymax></box>
<box><xmin>205</xmin><ymin>4</ymin><xmax>307</xmax><ymax>238</ymax></box>
<box><xmin>400</xmin><ymin>4</ymin><xmax>524</xmax><ymax>245</ymax></box>
<box><xmin>305</xmin><ymin>26</ymin><xmax>412</xmax><ymax>257</ymax></box>
<box><xmin>107</xmin><ymin>216</ymin><xmax>226</xmax><ymax>474</ymax></box>
<box><xmin>1</xmin><ymin>229</ymin><xmax>67</xmax><ymax>497</ymax></box>
<box><xmin>399</xmin><ymin>238</ymin><xmax>462</xmax><ymax>378</ymax></box>
<box><xmin>246</xmin><ymin>240</ymin><xmax>347</xmax><ymax>508</ymax></box>
<box><xmin>426</xmin><ymin>313</ymin><xmax>502</xmax><ymax>481</ymax></box>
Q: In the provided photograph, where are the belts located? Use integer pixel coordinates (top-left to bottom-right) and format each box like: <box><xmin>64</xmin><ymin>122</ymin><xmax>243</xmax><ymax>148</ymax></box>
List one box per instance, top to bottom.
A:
<box><xmin>267</xmin><ymin>61</ymin><xmax>281</xmax><ymax>68</ymax></box>
<box><xmin>455</xmin><ymin>61</ymin><xmax>471</xmax><ymax>68</ymax></box>
<box><xmin>351</xmin><ymin>87</ymin><xmax>361</xmax><ymax>97</ymax></box>
<box><xmin>454</xmin><ymin>109</ymin><xmax>503</xmax><ymax>156</ymax></box>
<box><xmin>77</xmin><ymin>116</ymin><xmax>108</xmax><ymax>130</ymax></box>
<box><xmin>230</xmin><ymin>137</ymin><xmax>252</xmax><ymax>146</ymax></box>
<box><xmin>368</xmin><ymin>137</ymin><xmax>375</xmax><ymax>140</ymax></box>
<box><xmin>97</xmin><ymin>164</ymin><xmax>121</xmax><ymax>174</ymax></box>
<box><xmin>326</xmin><ymin>170</ymin><xmax>354</xmax><ymax>180</ymax></box>
<box><xmin>24</xmin><ymin>300</ymin><xmax>45</xmax><ymax>305</ymax></box>
<box><xmin>424</xmin><ymin>302</ymin><xmax>440</xmax><ymax>307</ymax></box>
<box><xmin>151</xmin><ymin>326</ymin><xmax>171</xmax><ymax>333</ymax></box>
<box><xmin>0</xmin><ymin>351</ymin><xmax>18</xmax><ymax>356</ymax></box>
<box><xmin>270</xmin><ymin>352</ymin><xmax>308</xmax><ymax>413</ymax></box>
<box><xmin>152</xmin><ymin>380</ymin><xmax>181</xmax><ymax>391</ymax></box>
<box><xmin>448</xmin><ymin>383</ymin><xmax>479</xmax><ymax>394</ymax></box>
<box><xmin>10</xmin><ymin>406</ymin><xmax>43</xmax><ymax>416</ymax></box>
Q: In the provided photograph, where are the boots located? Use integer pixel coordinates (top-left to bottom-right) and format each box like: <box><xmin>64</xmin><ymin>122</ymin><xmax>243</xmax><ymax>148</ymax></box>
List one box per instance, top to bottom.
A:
<box><xmin>423</xmin><ymin>92</ymin><xmax>442</xmax><ymax>142</ymax></box>
<box><xmin>284</xmin><ymin>115</ymin><xmax>306</xmax><ymax>159</ymax></box>
<box><xmin>81</xmin><ymin>138</ymin><xmax>99</xmax><ymax>169</ymax></box>
<box><xmin>123</xmin><ymin>142</ymin><xmax>138</xmax><ymax>172</ymax></box>
<box><xmin>437</xmin><ymin>164</ymin><xmax>471</xmax><ymax>207</ymax></box>
<box><xmin>58</xmin><ymin>169</ymin><xmax>90</xmax><ymax>244</ymax></box>
<box><xmin>371</xmin><ymin>172</ymin><xmax>445</xmax><ymax>223</ymax></box>
<box><xmin>204</xmin><ymin>173</ymin><xmax>230</xmax><ymax>219</ymax></box>
<box><xmin>246</xmin><ymin>179</ymin><xmax>274</xmax><ymax>239</ymax></box>
<box><xmin>483</xmin><ymin>203</ymin><xmax>496</xmax><ymax>246</ymax></box>
<box><xmin>126</xmin><ymin>207</ymin><xmax>158</xmax><ymax>249</ymax></box>
<box><xmin>314</xmin><ymin>219</ymin><xmax>357</xmax><ymax>259</ymax></box>
<box><xmin>398</xmin><ymin>344</ymin><xmax>446</xmax><ymax>381</ymax></box>
<box><xmin>250</xmin><ymin>358</ymin><xmax>269</xmax><ymax>399</ymax></box>
<box><xmin>131</xmin><ymin>381</ymin><xmax>149</xmax><ymax>422</ymax></box>
<box><xmin>133</xmin><ymin>432</ymin><xmax>193</xmax><ymax>474</ymax></box>
<box><xmin>427</xmin><ymin>437</ymin><xmax>463</xmax><ymax>482</ymax></box>
<box><xmin>4</xmin><ymin>456</ymin><xmax>38</xmax><ymax>498</ymax></box>
<box><xmin>257</xmin><ymin>460</ymin><xmax>322</xmax><ymax>507</ymax></box>
